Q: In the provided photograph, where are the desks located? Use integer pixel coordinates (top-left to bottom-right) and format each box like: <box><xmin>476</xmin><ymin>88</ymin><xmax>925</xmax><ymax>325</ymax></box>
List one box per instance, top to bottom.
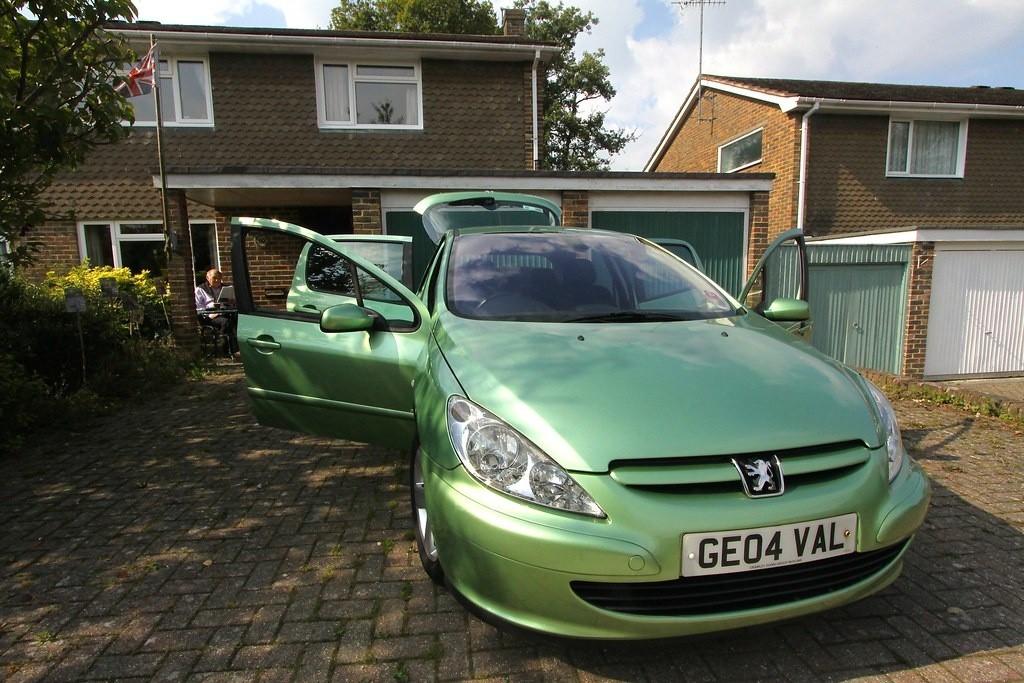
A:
<box><xmin>201</xmin><ymin>309</ymin><xmax>238</xmax><ymax>354</ymax></box>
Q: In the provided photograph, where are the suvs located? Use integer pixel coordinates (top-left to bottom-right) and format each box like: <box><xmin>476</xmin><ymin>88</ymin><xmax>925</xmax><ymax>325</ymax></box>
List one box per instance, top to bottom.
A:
<box><xmin>230</xmin><ymin>188</ymin><xmax>931</xmax><ymax>641</ymax></box>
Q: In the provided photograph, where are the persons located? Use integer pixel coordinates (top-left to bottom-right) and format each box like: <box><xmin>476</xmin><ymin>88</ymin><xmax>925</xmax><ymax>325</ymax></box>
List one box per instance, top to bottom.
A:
<box><xmin>194</xmin><ymin>269</ymin><xmax>239</xmax><ymax>358</ymax></box>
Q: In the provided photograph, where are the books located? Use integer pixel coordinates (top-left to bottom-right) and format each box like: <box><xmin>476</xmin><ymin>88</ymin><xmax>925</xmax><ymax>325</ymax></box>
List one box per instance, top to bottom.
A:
<box><xmin>217</xmin><ymin>285</ymin><xmax>236</xmax><ymax>306</ymax></box>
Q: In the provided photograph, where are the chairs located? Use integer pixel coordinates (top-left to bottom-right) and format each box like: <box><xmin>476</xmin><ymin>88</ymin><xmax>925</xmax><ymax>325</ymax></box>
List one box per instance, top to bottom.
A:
<box><xmin>457</xmin><ymin>259</ymin><xmax>502</xmax><ymax>315</ymax></box>
<box><xmin>549</xmin><ymin>259</ymin><xmax>615</xmax><ymax>313</ymax></box>
<box><xmin>198</xmin><ymin>313</ymin><xmax>232</xmax><ymax>358</ymax></box>
